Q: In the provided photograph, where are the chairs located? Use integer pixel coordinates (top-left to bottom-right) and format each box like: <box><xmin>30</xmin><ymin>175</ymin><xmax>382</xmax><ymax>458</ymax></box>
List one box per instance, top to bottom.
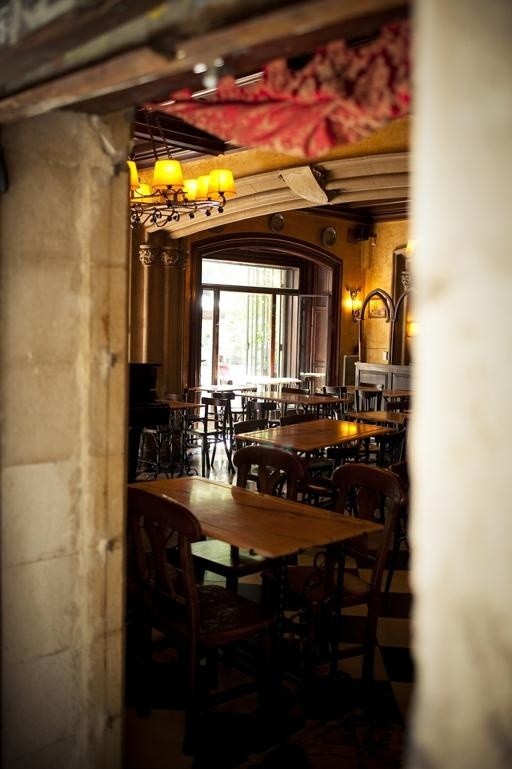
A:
<box><xmin>234</xmin><ymin>382</ymin><xmax>408</xmax><ymax>514</ymax></box>
<box><xmin>129</xmin><ymin>391</ymin><xmax>234</xmax><ymax>477</ymax></box>
<box><xmin>128</xmin><ymin>443</ymin><xmax>403</xmax><ymax>758</ymax></box>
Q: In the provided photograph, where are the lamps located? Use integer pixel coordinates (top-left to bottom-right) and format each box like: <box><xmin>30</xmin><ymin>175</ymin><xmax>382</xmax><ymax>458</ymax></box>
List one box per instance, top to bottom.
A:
<box><xmin>127</xmin><ymin>104</ymin><xmax>236</xmax><ymax>228</ymax></box>
<box><xmin>350</xmin><ymin>290</ymin><xmax>360</xmax><ymax>323</ymax></box>
